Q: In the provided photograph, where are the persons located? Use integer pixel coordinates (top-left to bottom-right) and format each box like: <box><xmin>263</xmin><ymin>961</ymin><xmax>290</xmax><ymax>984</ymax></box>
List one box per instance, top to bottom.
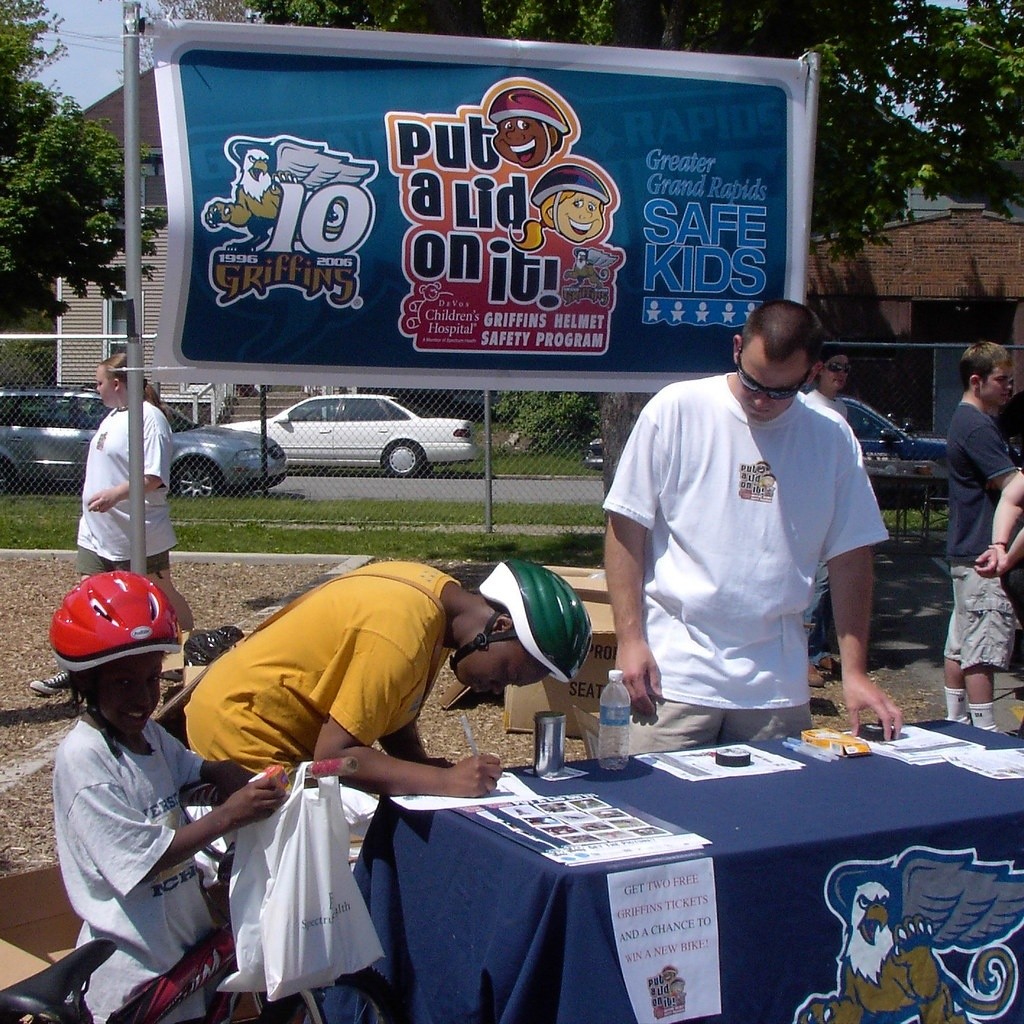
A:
<box><xmin>600</xmin><ymin>300</ymin><xmax>904</xmax><ymax>744</ymax></box>
<box><xmin>942</xmin><ymin>342</ymin><xmax>1024</xmax><ymax>731</ymax></box>
<box><xmin>802</xmin><ymin>343</ymin><xmax>849</xmax><ymax>685</ymax></box>
<box><xmin>31</xmin><ymin>350</ymin><xmax>194</xmax><ymax>693</ymax></box>
<box><xmin>973</xmin><ymin>472</ymin><xmax>1024</xmax><ymax>578</ymax></box>
<box><xmin>182</xmin><ymin>559</ymin><xmax>593</xmax><ymax>796</ymax></box>
<box><xmin>51</xmin><ymin>569</ymin><xmax>288</xmax><ymax>1024</ymax></box>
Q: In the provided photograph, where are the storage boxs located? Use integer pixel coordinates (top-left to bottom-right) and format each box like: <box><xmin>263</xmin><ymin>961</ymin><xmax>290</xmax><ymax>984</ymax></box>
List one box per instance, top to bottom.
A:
<box><xmin>504</xmin><ymin>565</ymin><xmax>617</xmax><ymax>738</ymax></box>
<box><xmin>801</xmin><ymin>728</ymin><xmax>871</xmax><ymax>758</ymax></box>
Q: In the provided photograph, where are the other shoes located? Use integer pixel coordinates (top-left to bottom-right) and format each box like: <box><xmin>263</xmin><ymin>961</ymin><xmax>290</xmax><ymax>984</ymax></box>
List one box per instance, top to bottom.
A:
<box><xmin>808</xmin><ymin>662</ymin><xmax>825</xmax><ymax>687</ymax></box>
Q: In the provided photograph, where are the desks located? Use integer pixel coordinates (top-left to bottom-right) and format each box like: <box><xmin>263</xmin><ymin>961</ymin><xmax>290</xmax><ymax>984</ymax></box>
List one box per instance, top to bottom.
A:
<box><xmin>864</xmin><ymin>460</ymin><xmax>948</xmax><ymax>553</ymax></box>
<box><xmin>303</xmin><ymin>716</ymin><xmax>1024</xmax><ymax>1024</ymax></box>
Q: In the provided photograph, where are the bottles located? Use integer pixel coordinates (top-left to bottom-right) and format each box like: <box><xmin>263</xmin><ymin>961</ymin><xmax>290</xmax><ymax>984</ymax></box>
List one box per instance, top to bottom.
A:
<box><xmin>599</xmin><ymin>670</ymin><xmax>631</xmax><ymax>770</ymax></box>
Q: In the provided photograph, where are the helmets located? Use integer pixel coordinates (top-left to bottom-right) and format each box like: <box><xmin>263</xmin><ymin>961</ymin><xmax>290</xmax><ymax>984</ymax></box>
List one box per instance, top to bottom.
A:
<box><xmin>480</xmin><ymin>560</ymin><xmax>592</xmax><ymax>681</ymax></box>
<box><xmin>50</xmin><ymin>570</ymin><xmax>182</xmax><ymax>672</ymax></box>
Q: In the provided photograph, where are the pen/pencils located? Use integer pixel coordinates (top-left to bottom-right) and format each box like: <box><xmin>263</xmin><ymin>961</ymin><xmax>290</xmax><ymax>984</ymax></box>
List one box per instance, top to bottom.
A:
<box><xmin>461</xmin><ymin>715</ymin><xmax>492</xmax><ymax>795</ymax></box>
<box><xmin>786</xmin><ymin>737</ymin><xmax>839</xmax><ymax>761</ymax></box>
<box><xmin>782</xmin><ymin>742</ymin><xmax>832</xmax><ymax>763</ymax></box>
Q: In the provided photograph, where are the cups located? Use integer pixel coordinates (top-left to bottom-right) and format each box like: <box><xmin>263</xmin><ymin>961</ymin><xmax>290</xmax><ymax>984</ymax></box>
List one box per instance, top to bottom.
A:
<box><xmin>533</xmin><ymin>711</ymin><xmax>566</xmax><ymax>777</ymax></box>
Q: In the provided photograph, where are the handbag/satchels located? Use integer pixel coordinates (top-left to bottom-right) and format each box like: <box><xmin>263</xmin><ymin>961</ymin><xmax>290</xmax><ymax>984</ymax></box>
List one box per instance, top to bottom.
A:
<box><xmin>215</xmin><ymin>762</ymin><xmax>382</xmax><ymax>1002</ymax></box>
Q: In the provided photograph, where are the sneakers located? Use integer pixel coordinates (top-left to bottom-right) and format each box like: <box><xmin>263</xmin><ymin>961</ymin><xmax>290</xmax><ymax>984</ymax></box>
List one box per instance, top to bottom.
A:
<box><xmin>30</xmin><ymin>669</ymin><xmax>71</xmax><ymax>694</ymax></box>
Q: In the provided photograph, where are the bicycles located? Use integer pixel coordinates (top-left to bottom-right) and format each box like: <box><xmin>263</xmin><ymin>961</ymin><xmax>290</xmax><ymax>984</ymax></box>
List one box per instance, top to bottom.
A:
<box><xmin>0</xmin><ymin>754</ymin><xmax>361</xmax><ymax>1024</ymax></box>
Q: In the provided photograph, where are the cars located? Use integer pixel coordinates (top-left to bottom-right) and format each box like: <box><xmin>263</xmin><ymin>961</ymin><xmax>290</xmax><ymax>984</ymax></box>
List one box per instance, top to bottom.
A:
<box><xmin>0</xmin><ymin>385</ymin><xmax>290</xmax><ymax>499</ymax></box>
<box><xmin>833</xmin><ymin>390</ymin><xmax>1023</xmax><ymax>514</ymax></box>
<box><xmin>217</xmin><ymin>393</ymin><xmax>476</xmax><ymax>479</ymax></box>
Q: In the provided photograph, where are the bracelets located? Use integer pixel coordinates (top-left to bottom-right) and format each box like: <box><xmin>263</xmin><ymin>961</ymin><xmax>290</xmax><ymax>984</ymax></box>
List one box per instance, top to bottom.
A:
<box><xmin>994</xmin><ymin>542</ymin><xmax>1007</xmax><ymax>546</ymax></box>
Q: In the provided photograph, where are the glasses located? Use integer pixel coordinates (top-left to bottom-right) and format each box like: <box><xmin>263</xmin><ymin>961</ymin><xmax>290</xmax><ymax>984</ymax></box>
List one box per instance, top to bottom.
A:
<box><xmin>735</xmin><ymin>340</ymin><xmax>812</xmax><ymax>400</ymax></box>
<box><xmin>825</xmin><ymin>362</ymin><xmax>850</xmax><ymax>373</ymax></box>
